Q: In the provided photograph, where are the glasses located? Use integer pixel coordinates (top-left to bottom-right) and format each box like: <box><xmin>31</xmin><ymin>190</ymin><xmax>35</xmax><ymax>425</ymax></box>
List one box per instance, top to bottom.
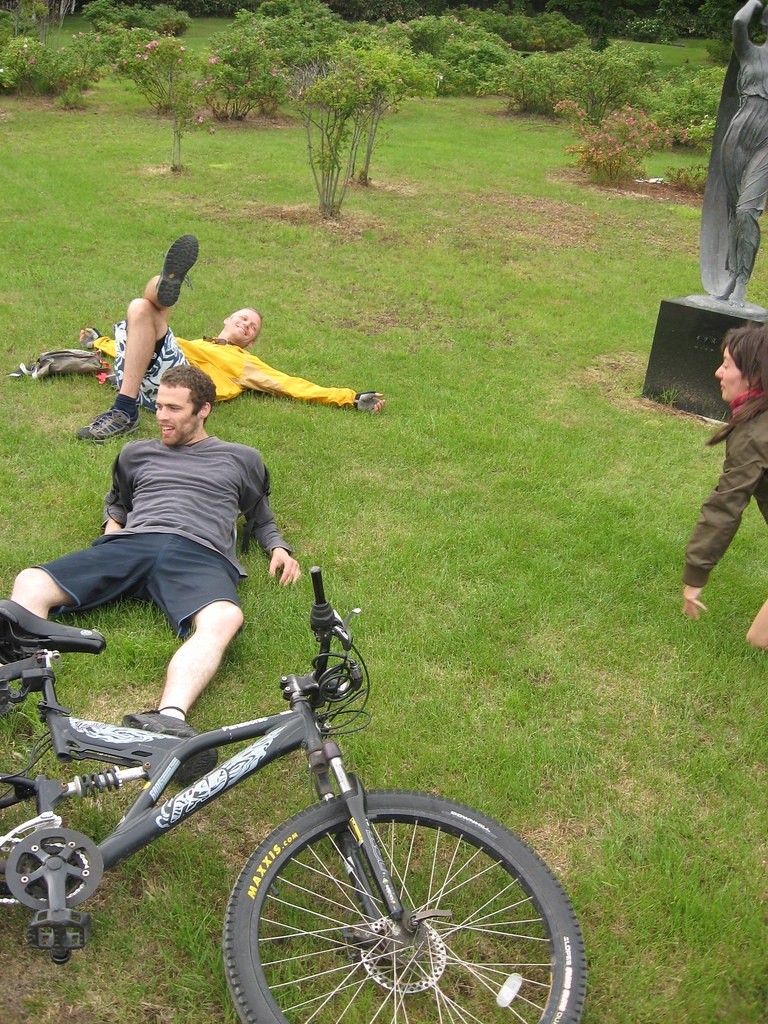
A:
<box><xmin>203</xmin><ymin>336</ymin><xmax>237</xmax><ymax>345</ymax></box>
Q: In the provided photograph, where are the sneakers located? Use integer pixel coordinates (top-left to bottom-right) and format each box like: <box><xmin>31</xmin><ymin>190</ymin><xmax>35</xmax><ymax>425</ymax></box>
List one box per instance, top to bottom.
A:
<box><xmin>156</xmin><ymin>234</ymin><xmax>199</xmax><ymax>307</ymax></box>
<box><xmin>76</xmin><ymin>405</ymin><xmax>141</xmax><ymax>442</ymax></box>
<box><xmin>121</xmin><ymin>710</ymin><xmax>218</xmax><ymax>784</ymax></box>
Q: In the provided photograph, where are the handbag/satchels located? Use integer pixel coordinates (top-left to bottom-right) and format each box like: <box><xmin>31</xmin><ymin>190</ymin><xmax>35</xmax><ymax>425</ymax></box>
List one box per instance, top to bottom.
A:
<box><xmin>5</xmin><ymin>348</ymin><xmax>118</xmax><ymax>387</ymax></box>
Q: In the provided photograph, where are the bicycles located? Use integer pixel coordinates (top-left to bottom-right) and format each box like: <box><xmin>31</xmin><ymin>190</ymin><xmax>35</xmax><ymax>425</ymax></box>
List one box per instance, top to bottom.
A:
<box><xmin>0</xmin><ymin>566</ymin><xmax>588</xmax><ymax>1024</ymax></box>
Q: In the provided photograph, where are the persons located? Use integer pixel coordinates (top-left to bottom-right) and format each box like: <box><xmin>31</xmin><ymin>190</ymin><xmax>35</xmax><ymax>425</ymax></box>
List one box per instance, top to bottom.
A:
<box><xmin>700</xmin><ymin>0</ymin><xmax>768</xmax><ymax>308</ymax></box>
<box><xmin>76</xmin><ymin>233</ymin><xmax>386</xmax><ymax>444</ymax></box>
<box><xmin>680</xmin><ymin>323</ymin><xmax>768</xmax><ymax>651</ymax></box>
<box><xmin>0</xmin><ymin>364</ymin><xmax>301</xmax><ymax>790</ymax></box>
